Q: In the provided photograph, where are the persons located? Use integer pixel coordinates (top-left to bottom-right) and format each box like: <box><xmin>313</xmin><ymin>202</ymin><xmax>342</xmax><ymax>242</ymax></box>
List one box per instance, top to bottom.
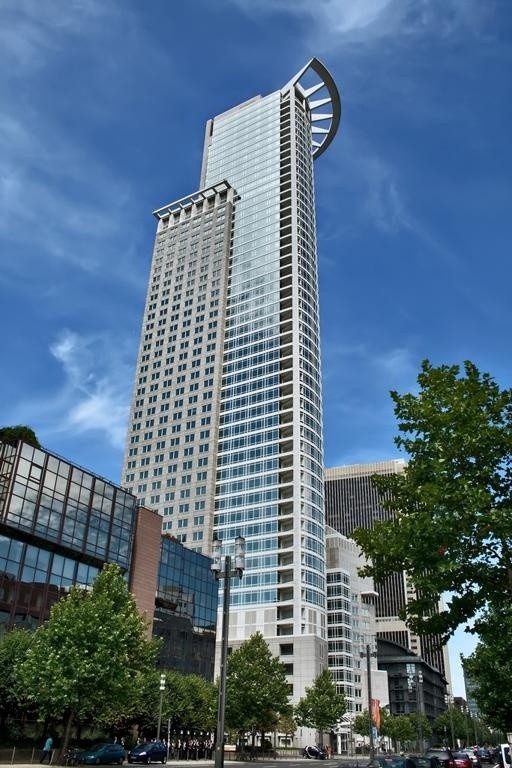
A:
<box><xmin>39</xmin><ymin>735</ymin><xmax>54</xmax><ymax>765</ymax></box>
<box><xmin>114</xmin><ymin>736</ymin><xmax>126</xmax><ymax>747</ymax></box>
<box><xmin>136</xmin><ymin>737</ymin><xmax>214</xmax><ymax>761</ymax></box>
<box><xmin>325</xmin><ymin>744</ymin><xmax>331</xmax><ymax>760</ymax></box>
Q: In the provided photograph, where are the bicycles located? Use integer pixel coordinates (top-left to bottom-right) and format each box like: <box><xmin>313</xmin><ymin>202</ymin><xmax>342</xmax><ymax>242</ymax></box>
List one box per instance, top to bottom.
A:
<box><xmin>62</xmin><ymin>747</ymin><xmax>79</xmax><ymax>766</ymax></box>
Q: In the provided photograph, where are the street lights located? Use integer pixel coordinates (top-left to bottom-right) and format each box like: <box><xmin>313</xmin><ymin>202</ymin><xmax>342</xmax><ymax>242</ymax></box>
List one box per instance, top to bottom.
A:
<box><xmin>210</xmin><ymin>537</ymin><xmax>245</xmax><ymax>768</ymax></box>
<box><xmin>358</xmin><ymin>633</ymin><xmax>378</xmax><ymax>761</ymax></box>
<box><xmin>408</xmin><ymin>670</ymin><xmax>423</xmax><ymax>752</ymax></box>
<box><xmin>156</xmin><ymin>674</ymin><xmax>166</xmax><ymax>743</ymax></box>
<box><xmin>444</xmin><ymin>694</ymin><xmax>455</xmax><ymax>750</ymax></box>
<box><xmin>469</xmin><ymin>710</ymin><xmax>478</xmax><ymax>745</ymax></box>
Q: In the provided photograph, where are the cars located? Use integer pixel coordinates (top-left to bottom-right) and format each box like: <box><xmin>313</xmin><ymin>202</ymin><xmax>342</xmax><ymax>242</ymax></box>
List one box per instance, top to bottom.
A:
<box><xmin>127</xmin><ymin>742</ymin><xmax>167</xmax><ymax>765</ymax></box>
<box><xmin>78</xmin><ymin>743</ymin><xmax>126</xmax><ymax>765</ymax></box>
<box><xmin>368</xmin><ymin>743</ymin><xmax>512</xmax><ymax>768</ymax></box>
<box><xmin>303</xmin><ymin>745</ymin><xmax>325</xmax><ymax>760</ymax></box>
